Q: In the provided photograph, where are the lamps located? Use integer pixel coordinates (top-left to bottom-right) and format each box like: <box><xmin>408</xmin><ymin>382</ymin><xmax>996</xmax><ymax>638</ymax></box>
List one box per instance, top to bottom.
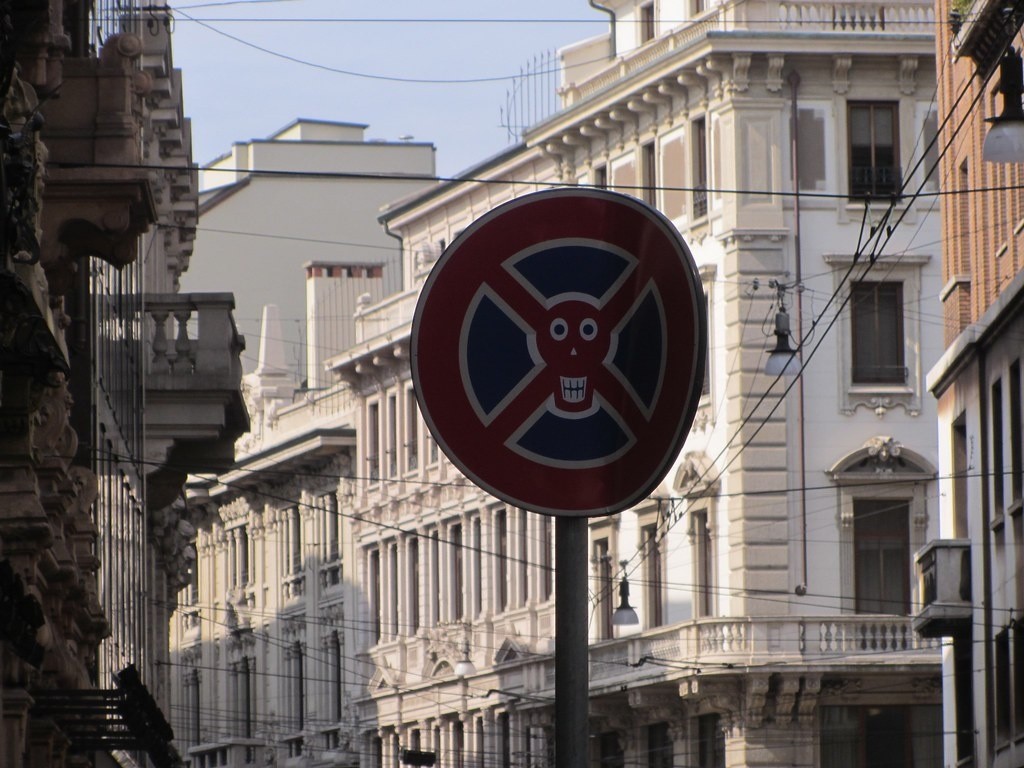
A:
<box><xmin>982</xmin><ymin>46</ymin><xmax>1024</xmax><ymax>162</ymax></box>
<box><xmin>763</xmin><ymin>306</ymin><xmax>802</xmax><ymax>376</ymax></box>
<box><xmin>610</xmin><ymin>573</ymin><xmax>639</xmax><ymax>624</ymax></box>
<box><xmin>454</xmin><ymin>638</ymin><xmax>477</xmax><ymax>675</ymax></box>
